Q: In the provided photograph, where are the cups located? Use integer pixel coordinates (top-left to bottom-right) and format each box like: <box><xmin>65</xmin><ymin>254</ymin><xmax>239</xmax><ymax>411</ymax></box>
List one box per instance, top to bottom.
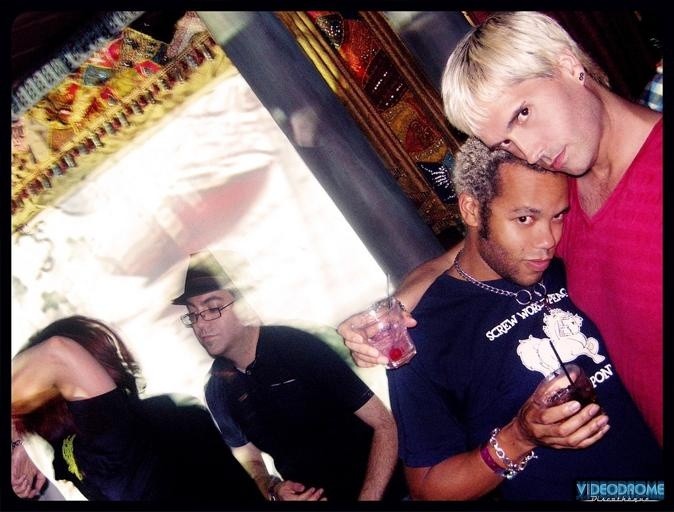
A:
<box><xmin>353</xmin><ymin>300</ymin><xmax>420</xmax><ymax>370</ymax></box>
<box><xmin>535</xmin><ymin>366</ymin><xmax>608</xmax><ymax>427</ymax></box>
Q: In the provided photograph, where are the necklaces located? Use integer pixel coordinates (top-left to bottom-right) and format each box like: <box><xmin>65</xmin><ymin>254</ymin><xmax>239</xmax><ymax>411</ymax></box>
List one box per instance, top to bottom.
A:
<box><xmin>452</xmin><ymin>247</ymin><xmax>547</xmax><ymax>306</ymax></box>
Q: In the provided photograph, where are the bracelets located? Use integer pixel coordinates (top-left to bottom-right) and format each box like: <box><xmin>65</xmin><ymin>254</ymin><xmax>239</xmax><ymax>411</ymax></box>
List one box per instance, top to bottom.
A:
<box><xmin>488</xmin><ymin>426</ymin><xmax>536</xmax><ymax>471</ymax></box>
<box><xmin>9</xmin><ymin>440</ymin><xmax>22</xmax><ymax>447</ymax></box>
<box><xmin>479</xmin><ymin>441</ymin><xmax>516</xmax><ymax>480</ymax></box>
<box><xmin>270</xmin><ymin>477</ymin><xmax>279</xmax><ymax>501</ymax></box>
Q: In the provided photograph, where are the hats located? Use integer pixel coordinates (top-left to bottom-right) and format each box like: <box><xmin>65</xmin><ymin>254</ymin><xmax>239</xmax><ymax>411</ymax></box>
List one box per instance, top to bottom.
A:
<box><xmin>171</xmin><ymin>251</ymin><xmax>255</xmax><ymax>306</ymax></box>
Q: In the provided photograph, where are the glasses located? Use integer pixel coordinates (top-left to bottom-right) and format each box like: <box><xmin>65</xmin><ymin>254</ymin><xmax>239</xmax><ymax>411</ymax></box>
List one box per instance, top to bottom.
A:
<box><xmin>179</xmin><ymin>297</ymin><xmax>240</xmax><ymax>327</ymax></box>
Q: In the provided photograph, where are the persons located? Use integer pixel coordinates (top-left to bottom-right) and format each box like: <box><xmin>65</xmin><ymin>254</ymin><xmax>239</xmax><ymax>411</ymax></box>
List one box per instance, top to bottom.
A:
<box><xmin>336</xmin><ymin>11</ymin><xmax>662</xmax><ymax>444</ymax></box>
<box><xmin>383</xmin><ymin>138</ymin><xmax>664</xmax><ymax>502</ymax></box>
<box><xmin>171</xmin><ymin>251</ymin><xmax>411</xmax><ymax>501</ymax></box>
<box><xmin>9</xmin><ymin>314</ymin><xmax>267</xmax><ymax>501</ymax></box>
